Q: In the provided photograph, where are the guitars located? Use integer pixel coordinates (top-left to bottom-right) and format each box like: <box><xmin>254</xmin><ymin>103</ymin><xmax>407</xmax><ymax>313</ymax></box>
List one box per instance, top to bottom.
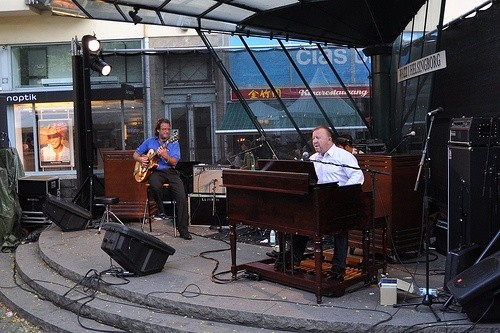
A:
<box><xmin>134</xmin><ymin>133</ymin><xmax>181</xmax><ymax>183</ymax></box>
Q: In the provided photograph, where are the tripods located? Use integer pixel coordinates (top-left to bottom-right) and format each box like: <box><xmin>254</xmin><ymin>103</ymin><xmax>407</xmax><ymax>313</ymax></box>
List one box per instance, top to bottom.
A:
<box><xmin>393</xmin><ymin>117</ymin><xmax>448</xmax><ymax>320</ymax></box>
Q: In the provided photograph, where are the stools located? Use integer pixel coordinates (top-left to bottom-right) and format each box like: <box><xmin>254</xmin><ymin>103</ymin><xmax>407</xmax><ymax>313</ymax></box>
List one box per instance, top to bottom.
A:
<box><xmin>141</xmin><ymin>181</ymin><xmax>181</xmax><ymax>237</ymax></box>
<box><xmin>93</xmin><ymin>197</ymin><xmax>125</xmax><ymax>233</ymax></box>
<box><xmin>374</xmin><ymin>216</ymin><xmax>389</xmax><ymax>276</ymax></box>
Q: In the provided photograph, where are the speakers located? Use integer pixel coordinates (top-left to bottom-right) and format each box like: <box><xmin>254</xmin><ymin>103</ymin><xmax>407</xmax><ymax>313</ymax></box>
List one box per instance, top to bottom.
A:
<box><xmin>434</xmin><ymin>146</ymin><xmax>500</xmax><ymax>256</ymax></box>
<box><xmin>194</xmin><ymin>164</ymin><xmax>235</xmax><ymax>196</ymax></box>
<box><xmin>44</xmin><ymin>195</ymin><xmax>92</xmax><ymax>230</ymax></box>
<box><xmin>102</xmin><ymin>223</ymin><xmax>176</xmax><ymax>276</ymax></box>
<box><xmin>445</xmin><ymin>251</ymin><xmax>500</xmax><ymax>324</ymax></box>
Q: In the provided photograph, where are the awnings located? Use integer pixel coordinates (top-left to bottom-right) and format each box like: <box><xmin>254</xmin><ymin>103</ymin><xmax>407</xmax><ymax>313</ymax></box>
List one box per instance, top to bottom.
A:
<box><xmin>214</xmin><ymin>97</ymin><xmax>368</xmax><ymax>134</ymax></box>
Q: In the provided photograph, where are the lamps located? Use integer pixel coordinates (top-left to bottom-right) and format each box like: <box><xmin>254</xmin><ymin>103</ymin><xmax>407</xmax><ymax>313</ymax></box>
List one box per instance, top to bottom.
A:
<box><xmin>129</xmin><ymin>6</ymin><xmax>143</xmax><ymax>25</ymax></box>
<box><xmin>82</xmin><ymin>35</ymin><xmax>112</xmax><ymax>77</ymax></box>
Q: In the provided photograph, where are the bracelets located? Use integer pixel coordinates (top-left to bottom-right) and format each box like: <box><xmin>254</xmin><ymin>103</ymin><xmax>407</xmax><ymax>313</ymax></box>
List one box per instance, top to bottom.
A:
<box><xmin>164</xmin><ymin>157</ymin><xmax>170</xmax><ymax>164</ymax></box>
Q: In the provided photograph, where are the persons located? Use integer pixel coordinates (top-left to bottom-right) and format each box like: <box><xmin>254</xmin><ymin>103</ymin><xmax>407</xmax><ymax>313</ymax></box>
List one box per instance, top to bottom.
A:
<box><xmin>133</xmin><ymin>118</ymin><xmax>192</xmax><ymax>240</ymax></box>
<box><xmin>40</xmin><ymin>123</ymin><xmax>70</xmax><ymax>162</ymax></box>
<box><xmin>278</xmin><ymin>125</ymin><xmax>364</xmax><ymax>281</ymax></box>
<box><xmin>335</xmin><ymin>134</ymin><xmax>364</xmax><ymax>155</ymax></box>
<box><xmin>239</xmin><ymin>140</ymin><xmax>256</xmax><ymax>170</ymax></box>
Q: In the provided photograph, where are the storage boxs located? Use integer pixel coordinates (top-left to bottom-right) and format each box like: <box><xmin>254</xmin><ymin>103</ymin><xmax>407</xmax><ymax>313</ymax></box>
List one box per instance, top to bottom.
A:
<box><xmin>17</xmin><ymin>176</ymin><xmax>60</xmax><ymax>227</ymax></box>
<box><xmin>187</xmin><ymin>192</ymin><xmax>230</xmax><ymax>229</ymax></box>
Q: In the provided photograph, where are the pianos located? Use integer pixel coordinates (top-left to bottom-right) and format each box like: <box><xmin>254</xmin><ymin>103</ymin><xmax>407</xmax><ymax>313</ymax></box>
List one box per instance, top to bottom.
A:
<box><xmin>222</xmin><ymin>159</ymin><xmax>373</xmax><ymax>303</ymax></box>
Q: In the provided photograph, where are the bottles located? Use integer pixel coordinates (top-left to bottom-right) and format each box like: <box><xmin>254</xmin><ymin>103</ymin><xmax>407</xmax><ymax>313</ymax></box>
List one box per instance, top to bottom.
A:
<box><xmin>270</xmin><ymin>230</ymin><xmax>276</xmax><ymax>247</ymax></box>
<box><xmin>418</xmin><ymin>288</ymin><xmax>438</xmax><ymax>297</ymax></box>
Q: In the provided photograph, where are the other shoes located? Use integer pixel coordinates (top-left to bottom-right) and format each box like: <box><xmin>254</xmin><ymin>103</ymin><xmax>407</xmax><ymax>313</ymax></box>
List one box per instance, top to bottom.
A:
<box><xmin>265</xmin><ymin>250</ymin><xmax>300</xmax><ymax>266</ymax></box>
<box><xmin>321</xmin><ymin>271</ymin><xmax>345</xmax><ymax>283</ymax></box>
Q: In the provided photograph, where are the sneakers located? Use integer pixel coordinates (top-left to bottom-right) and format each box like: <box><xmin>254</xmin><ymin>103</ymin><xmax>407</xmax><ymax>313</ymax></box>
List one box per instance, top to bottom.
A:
<box><xmin>154</xmin><ymin>213</ymin><xmax>168</xmax><ymax>220</ymax></box>
<box><xmin>179</xmin><ymin>232</ymin><xmax>192</xmax><ymax>240</ymax></box>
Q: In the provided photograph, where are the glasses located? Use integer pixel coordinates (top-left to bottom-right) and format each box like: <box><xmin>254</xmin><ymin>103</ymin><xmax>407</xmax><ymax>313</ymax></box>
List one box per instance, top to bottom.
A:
<box><xmin>162</xmin><ymin>128</ymin><xmax>172</xmax><ymax>131</ymax></box>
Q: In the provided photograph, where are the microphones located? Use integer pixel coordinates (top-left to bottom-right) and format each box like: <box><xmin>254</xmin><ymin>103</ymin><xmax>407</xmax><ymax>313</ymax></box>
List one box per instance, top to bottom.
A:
<box><xmin>427</xmin><ymin>106</ymin><xmax>443</xmax><ymax>117</ymax></box>
<box><xmin>402</xmin><ymin>131</ymin><xmax>416</xmax><ymax>139</ymax></box>
<box><xmin>255</xmin><ymin>136</ymin><xmax>272</xmax><ymax>141</ymax></box>
<box><xmin>300</xmin><ymin>151</ymin><xmax>309</xmax><ymax>161</ymax></box>
